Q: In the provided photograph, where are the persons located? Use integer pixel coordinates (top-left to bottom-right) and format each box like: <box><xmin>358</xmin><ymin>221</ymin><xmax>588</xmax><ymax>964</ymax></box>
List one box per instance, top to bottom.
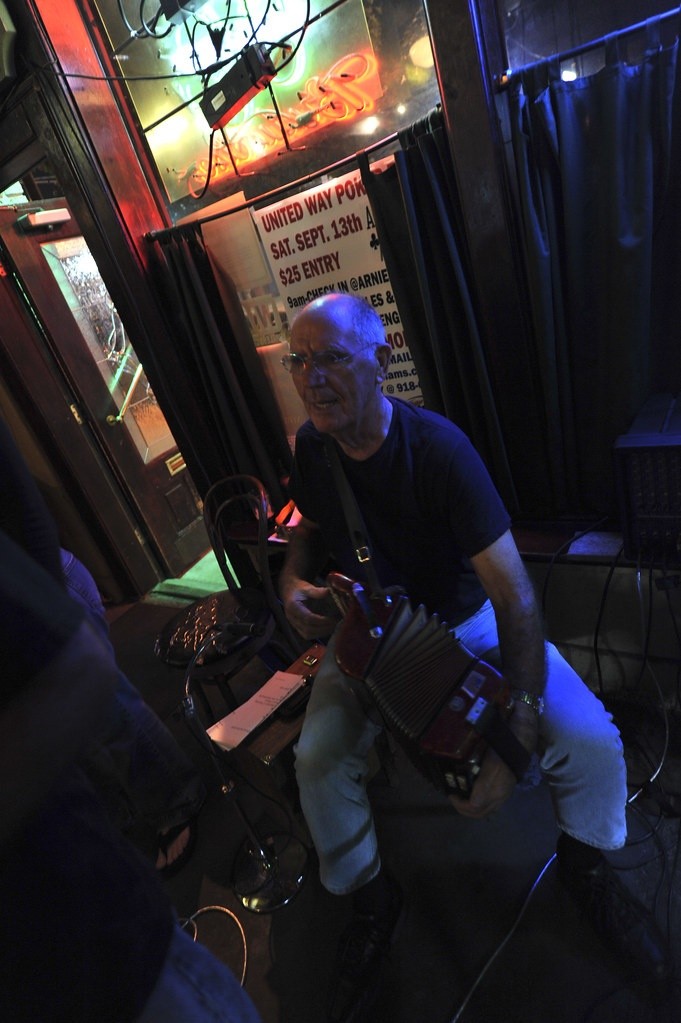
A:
<box><xmin>280</xmin><ymin>291</ymin><xmax>678</xmax><ymax>1023</ymax></box>
<box><xmin>0</xmin><ymin>416</ymin><xmax>263</xmax><ymax>1023</ymax></box>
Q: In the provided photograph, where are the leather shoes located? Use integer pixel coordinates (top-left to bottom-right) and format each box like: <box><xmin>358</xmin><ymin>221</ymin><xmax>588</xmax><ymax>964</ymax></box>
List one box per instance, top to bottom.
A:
<box><xmin>555</xmin><ymin>852</ymin><xmax>675</xmax><ymax>985</ymax></box>
<box><xmin>324</xmin><ymin>874</ymin><xmax>403</xmax><ymax>1023</ymax></box>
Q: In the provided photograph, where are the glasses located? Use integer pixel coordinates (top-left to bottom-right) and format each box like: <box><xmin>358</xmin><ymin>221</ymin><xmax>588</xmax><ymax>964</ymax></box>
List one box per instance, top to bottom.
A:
<box><xmin>280</xmin><ymin>342</ymin><xmax>380</xmax><ymax>373</ymax></box>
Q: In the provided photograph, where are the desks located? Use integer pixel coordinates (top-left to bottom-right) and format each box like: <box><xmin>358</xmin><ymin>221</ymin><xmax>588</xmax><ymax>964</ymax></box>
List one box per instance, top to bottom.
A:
<box><xmin>565</xmin><ymin>529</ymin><xmax>680</xmax><ymax>572</ymax></box>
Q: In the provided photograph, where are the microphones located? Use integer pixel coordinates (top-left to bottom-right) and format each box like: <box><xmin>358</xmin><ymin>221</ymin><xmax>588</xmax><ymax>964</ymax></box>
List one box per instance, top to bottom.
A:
<box><xmin>211</xmin><ymin>622</ymin><xmax>267</xmax><ymax>637</ymax></box>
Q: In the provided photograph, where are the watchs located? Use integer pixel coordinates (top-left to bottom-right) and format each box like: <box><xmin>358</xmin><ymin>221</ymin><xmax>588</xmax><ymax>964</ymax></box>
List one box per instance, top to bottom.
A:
<box><xmin>509</xmin><ymin>687</ymin><xmax>545</xmax><ymax>719</ymax></box>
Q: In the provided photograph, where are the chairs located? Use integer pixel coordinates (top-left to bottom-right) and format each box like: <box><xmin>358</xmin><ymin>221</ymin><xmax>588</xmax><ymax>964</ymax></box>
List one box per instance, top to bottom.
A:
<box><xmin>156</xmin><ymin>469</ymin><xmax>307</xmax><ymax>777</ymax></box>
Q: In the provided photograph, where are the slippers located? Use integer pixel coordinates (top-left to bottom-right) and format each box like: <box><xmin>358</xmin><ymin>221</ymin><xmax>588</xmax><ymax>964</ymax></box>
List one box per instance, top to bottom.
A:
<box><xmin>147</xmin><ymin>816</ymin><xmax>195</xmax><ymax>878</ymax></box>
